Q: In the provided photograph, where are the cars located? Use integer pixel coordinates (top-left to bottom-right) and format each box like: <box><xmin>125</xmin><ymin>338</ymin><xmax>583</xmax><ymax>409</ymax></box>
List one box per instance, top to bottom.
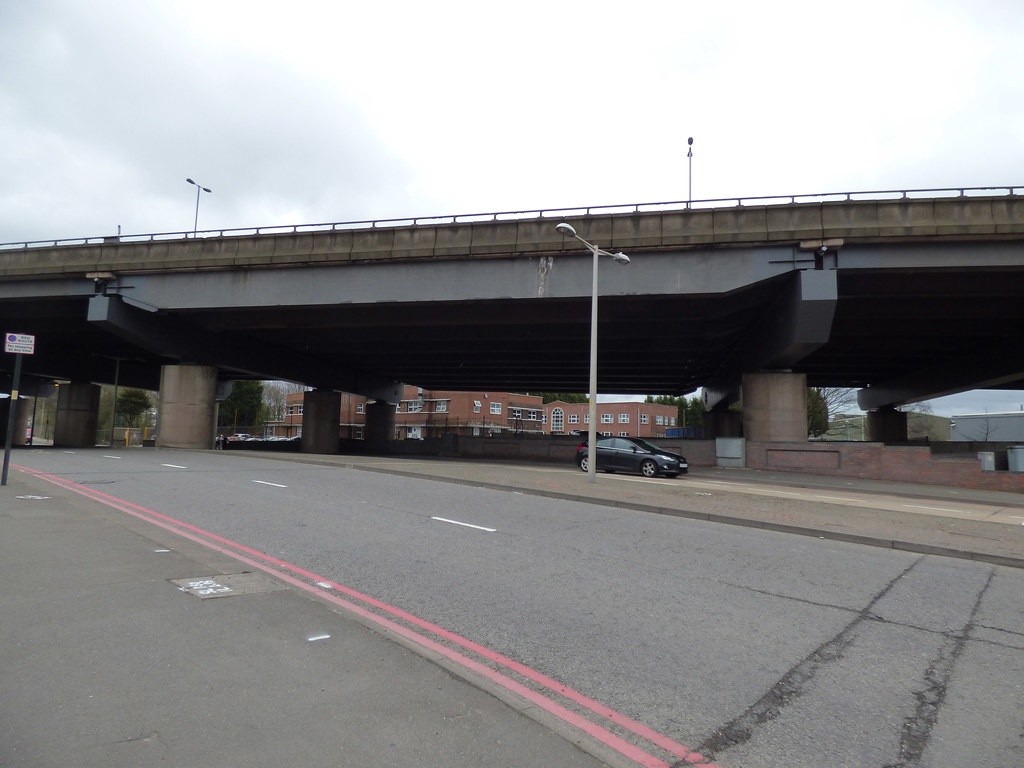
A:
<box><xmin>574</xmin><ymin>430</ymin><xmax>602</xmax><ymax>436</ymax></box>
<box><xmin>227</xmin><ymin>433</ymin><xmax>301</xmax><ymax>441</ymax></box>
<box><xmin>576</xmin><ymin>436</ymin><xmax>688</xmax><ymax>479</ymax></box>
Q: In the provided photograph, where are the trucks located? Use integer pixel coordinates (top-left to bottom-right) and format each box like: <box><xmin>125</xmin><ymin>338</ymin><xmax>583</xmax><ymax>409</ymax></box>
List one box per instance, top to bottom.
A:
<box><xmin>26</xmin><ymin>418</ymin><xmax>32</xmax><ymax>443</ymax></box>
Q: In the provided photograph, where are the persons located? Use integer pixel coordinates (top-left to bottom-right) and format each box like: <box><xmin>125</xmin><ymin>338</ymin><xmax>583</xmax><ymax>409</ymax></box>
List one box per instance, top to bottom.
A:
<box><xmin>412</xmin><ymin>428</ymin><xmax>417</xmax><ymax>439</ymax></box>
<box><xmin>216</xmin><ymin>435</ymin><xmax>229</xmax><ymax>450</ymax></box>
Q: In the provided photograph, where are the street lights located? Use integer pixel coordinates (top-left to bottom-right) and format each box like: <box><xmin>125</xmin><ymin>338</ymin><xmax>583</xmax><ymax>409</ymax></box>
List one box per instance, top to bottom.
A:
<box><xmin>687</xmin><ymin>137</ymin><xmax>693</xmax><ymax>208</ymax></box>
<box><xmin>187</xmin><ymin>179</ymin><xmax>211</xmax><ymax>238</ymax></box>
<box><xmin>556</xmin><ymin>222</ymin><xmax>631</xmax><ymax>475</ymax></box>
